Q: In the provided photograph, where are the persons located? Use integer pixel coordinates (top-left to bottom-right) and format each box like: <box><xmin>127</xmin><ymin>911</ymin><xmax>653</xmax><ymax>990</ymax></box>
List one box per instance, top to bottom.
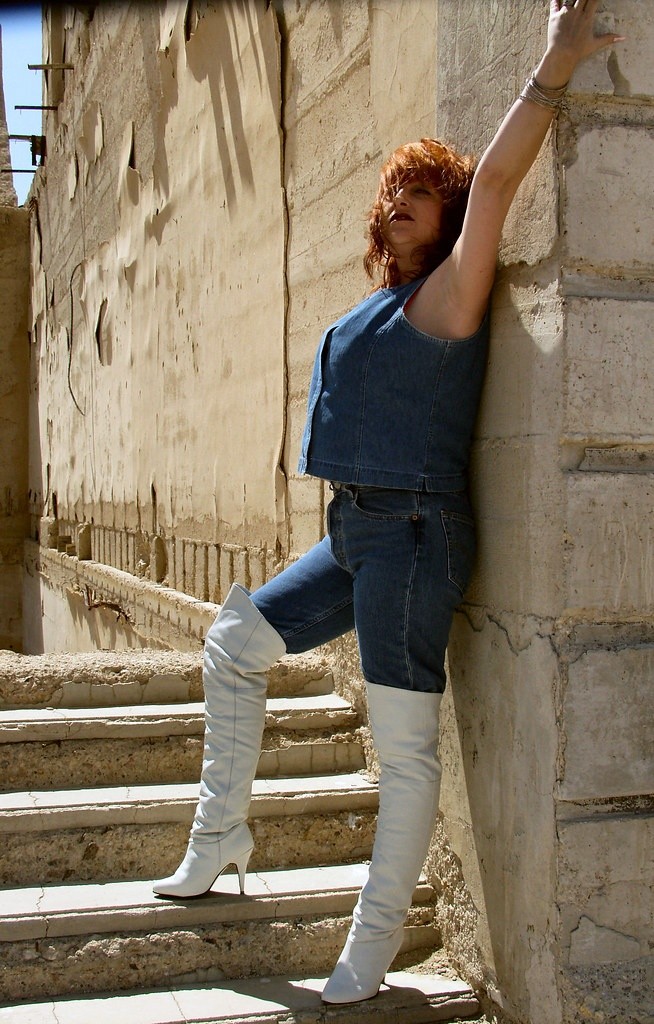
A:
<box><xmin>151</xmin><ymin>0</ymin><xmax>627</xmax><ymax>1008</ymax></box>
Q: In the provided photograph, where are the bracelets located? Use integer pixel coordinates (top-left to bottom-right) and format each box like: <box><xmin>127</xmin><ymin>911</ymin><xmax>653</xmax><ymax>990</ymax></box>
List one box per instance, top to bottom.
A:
<box><xmin>517</xmin><ymin>70</ymin><xmax>570</xmax><ymax>113</ymax></box>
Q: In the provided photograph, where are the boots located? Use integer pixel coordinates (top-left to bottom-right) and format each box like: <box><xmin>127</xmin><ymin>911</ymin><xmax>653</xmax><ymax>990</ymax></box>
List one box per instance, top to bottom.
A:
<box><xmin>153</xmin><ymin>583</ymin><xmax>287</xmax><ymax>898</ymax></box>
<box><xmin>321</xmin><ymin>679</ymin><xmax>443</xmax><ymax>1005</ymax></box>
